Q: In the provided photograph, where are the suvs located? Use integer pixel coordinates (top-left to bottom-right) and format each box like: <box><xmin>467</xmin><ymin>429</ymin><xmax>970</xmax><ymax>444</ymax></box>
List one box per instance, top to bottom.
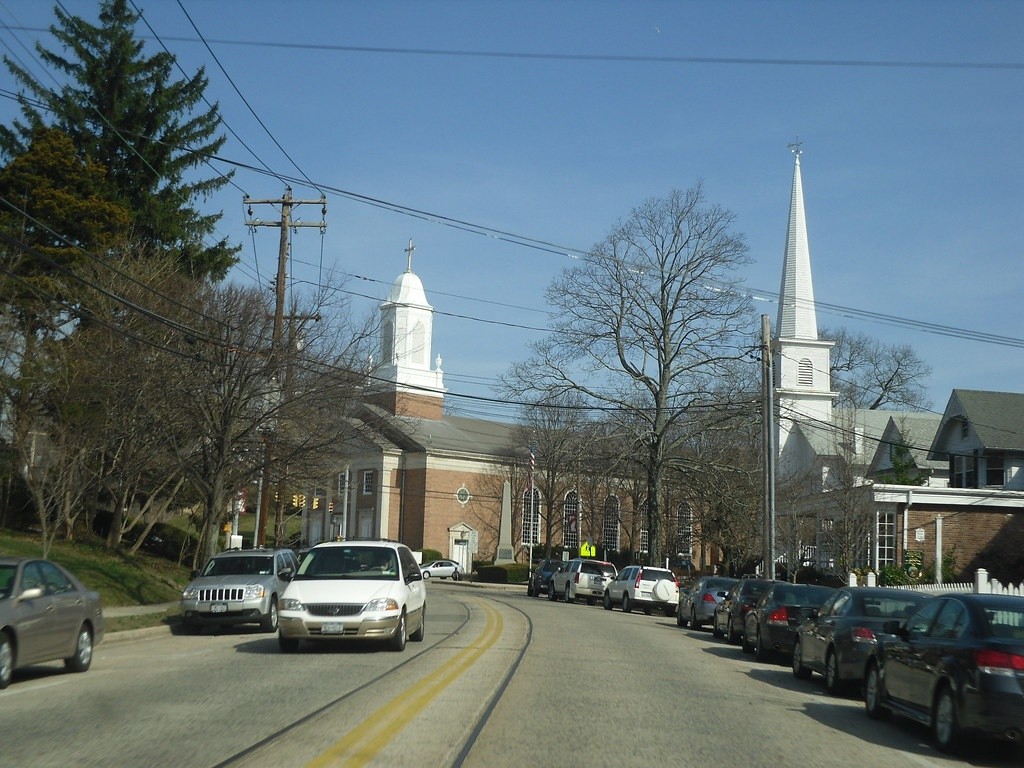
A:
<box><xmin>181</xmin><ymin>546</ymin><xmax>301</xmax><ymax>636</ymax></box>
<box><xmin>548</xmin><ymin>558</ymin><xmax>619</xmax><ymax>606</ymax></box>
<box><xmin>604</xmin><ymin>565</ymin><xmax>680</xmax><ymax>617</ymax></box>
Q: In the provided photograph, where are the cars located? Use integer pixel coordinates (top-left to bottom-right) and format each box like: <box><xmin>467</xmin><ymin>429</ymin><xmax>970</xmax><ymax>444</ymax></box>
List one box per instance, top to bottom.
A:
<box><xmin>276</xmin><ymin>540</ymin><xmax>427</xmax><ymax>654</ymax></box>
<box><xmin>0</xmin><ymin>556</ymin><xmax>105</xmax><ymax>688</ymax></box>
<box><xmin>420</xmin><ymin>559</ymin><xmax>463</xmax><ymax>581</ymax></box>
<box><xmin>527</xmin><ymin>559</ymin><xmax>565</xmax><ymax>598</ymax></box>
<box><xmin>661</xmin><ymin>560</ymin><xmax>696</xmax><ymax>572</ymax></box>
<box><xmin>677</xmin><ymin>576</ymin><xmax>741</xmax><ymax>630</ymax></box>
<box><xmin>713</xmin><ymin>579</ymin><xmax>791</xmax><ymax>644</ymax></box>
<box><xmin>744</xmin><ymin>583</ymin><xmax>839</xmax><ymax>663</ymax></box>
<box><xmin>793</xmin><ymin>586</ymin><xmax>933</xmax><ymax>696</ymax></box>
<box><xmin>865</xmin><ymin>593</ymin><xmax>1024</xmax><ymax>755</ymax></box>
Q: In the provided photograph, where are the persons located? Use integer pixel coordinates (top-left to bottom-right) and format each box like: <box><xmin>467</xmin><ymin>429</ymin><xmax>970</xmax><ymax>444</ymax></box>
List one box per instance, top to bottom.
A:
<box><xmin>377</xmin><ymin>553</ymin><xmax>394</xmax><ymax>571</ymax></box>
